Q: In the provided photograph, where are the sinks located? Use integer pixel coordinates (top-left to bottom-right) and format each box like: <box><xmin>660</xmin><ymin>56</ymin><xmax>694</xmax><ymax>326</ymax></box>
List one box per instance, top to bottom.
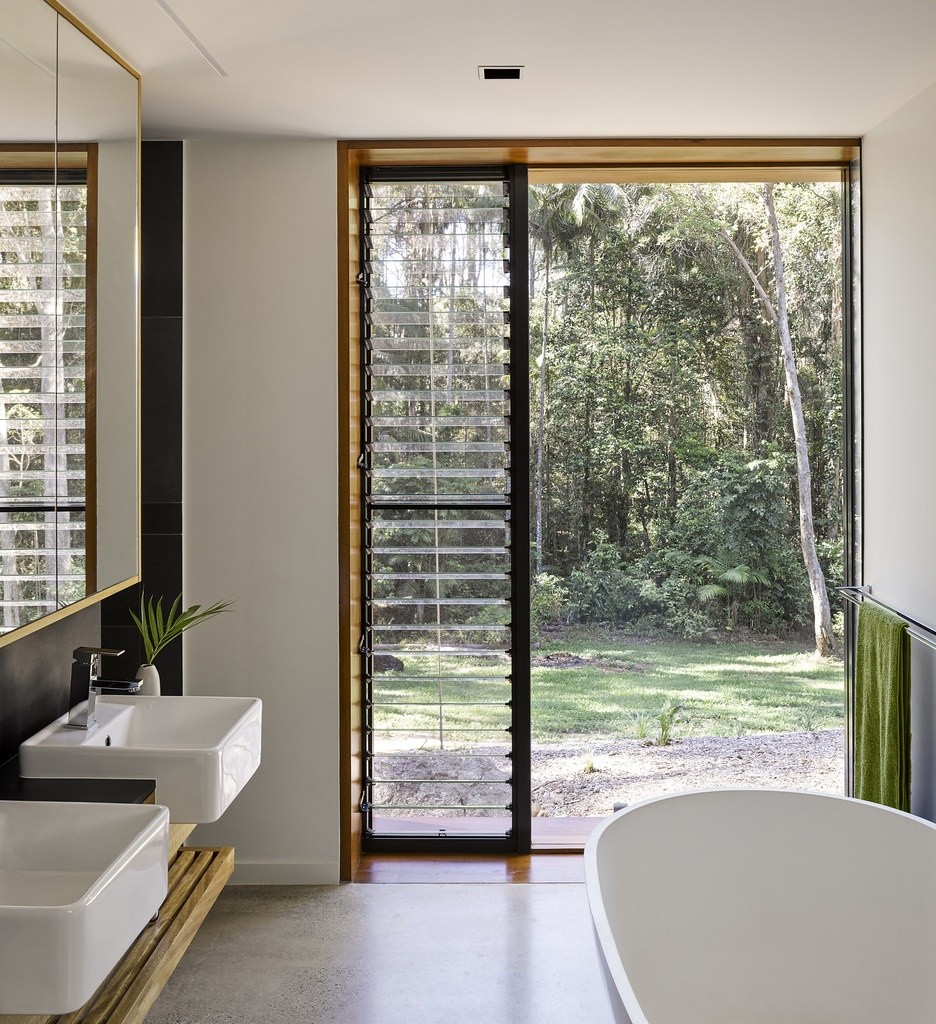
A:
<box><xmin>18</xmin><ymin>694</ymin><xmax>263</xmax><ymax>825</ymax></box>
<box><xmin>0</xmin><ymin>799</ymin><xmax>171</xmax><ymax>1017</ymax></box>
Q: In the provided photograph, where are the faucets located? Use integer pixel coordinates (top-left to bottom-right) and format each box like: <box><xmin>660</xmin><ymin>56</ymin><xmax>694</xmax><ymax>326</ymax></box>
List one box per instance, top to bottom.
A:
<box><xmin>64</xmin><ymin>645</ymin><xmax>144</xmax><ymax>731</ymax></box>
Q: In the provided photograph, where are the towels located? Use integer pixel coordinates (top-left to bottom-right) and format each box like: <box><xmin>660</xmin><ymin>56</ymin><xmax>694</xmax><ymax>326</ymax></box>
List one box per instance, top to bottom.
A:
<box><xmin>853</xmin><ymin>600</ymin><xmax>913</xmax><ymax>815</ymax></box>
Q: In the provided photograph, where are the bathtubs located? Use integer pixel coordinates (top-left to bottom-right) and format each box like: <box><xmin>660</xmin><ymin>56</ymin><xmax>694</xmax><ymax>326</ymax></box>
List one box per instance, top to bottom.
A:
<box><xmin>582</xmin><ymin>786</ymin><xmax>936</xmax><ymax>1024</ymax></box>
